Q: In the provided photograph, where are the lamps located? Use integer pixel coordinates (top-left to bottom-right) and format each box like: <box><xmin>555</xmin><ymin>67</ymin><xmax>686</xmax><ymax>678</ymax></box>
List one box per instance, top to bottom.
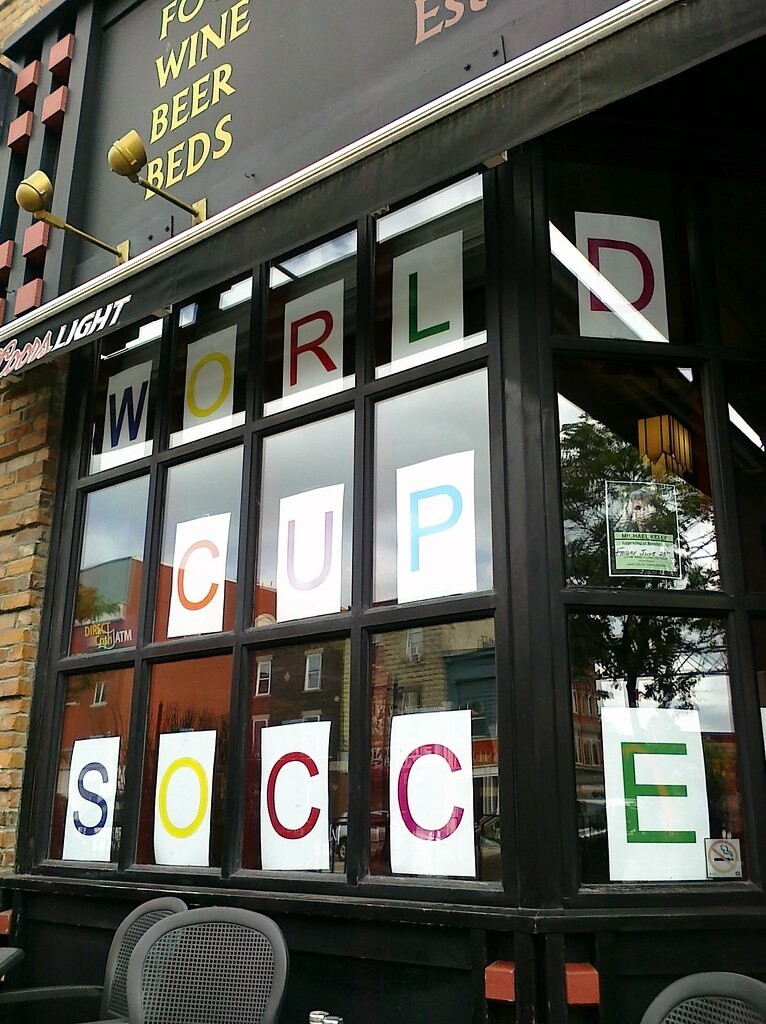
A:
<box><xmin>107</xmin><ymin>128</ymin><xmax>207</xmax><ymax>227</ymax></box>
<box><xmin>14</xmin><ymin>169</ymin><xmax>130</xmax><ymax>268</ymax></box>
<box><xmin>638</xmin><ymin>415</ymin><xmax>692</xmax><ymax>477</ymax></box>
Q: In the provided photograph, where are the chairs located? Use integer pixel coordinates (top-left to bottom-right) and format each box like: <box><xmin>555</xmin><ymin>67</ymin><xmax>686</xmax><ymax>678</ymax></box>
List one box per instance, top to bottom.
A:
<box><xmin>0</xmin><ymin>896</ymin><xmax>188</xmax><ymax>1024</ymax></box>
<box><xmin>125</xmin><ymin>906</ymin><xmax>287</xmax><ymax>1024</ymax></box>
<box><xmin>643</xmin><ymin>971</ymin><xmax>766</xmax><ymax>1024</ymax></box>
